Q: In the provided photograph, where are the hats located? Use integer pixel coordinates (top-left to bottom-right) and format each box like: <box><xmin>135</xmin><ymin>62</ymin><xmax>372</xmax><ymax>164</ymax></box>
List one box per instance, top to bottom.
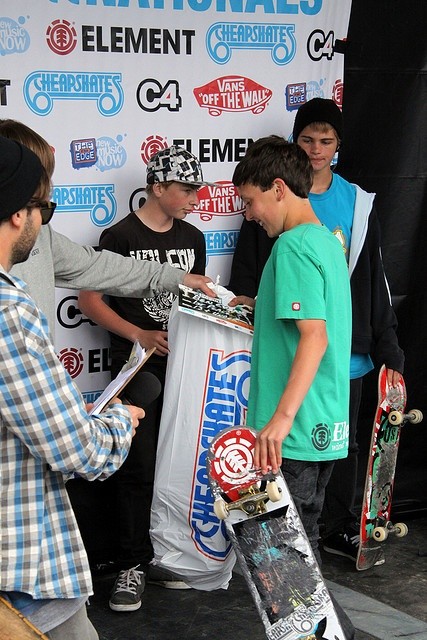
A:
<box><xmin>293</xmin><ymin>98</ymin><xmax>343</xmax><ymax>145</ymax></box>
<box><xmin>0</xmin><ymin>140</ymin><xmax>43</xmax><ymax>218</ymax></box>
<box><xmin>147</xmin><ymin>146</ymin><xmax>216</xmax><ymax>186</ymax></box>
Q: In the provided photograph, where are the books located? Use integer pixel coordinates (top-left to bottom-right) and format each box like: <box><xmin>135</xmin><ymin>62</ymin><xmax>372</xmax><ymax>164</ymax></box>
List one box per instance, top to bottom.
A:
<box><xmin>178</xmin><ymin>283</ymin><xmax>254</xmax><ymax>337</ymax></box>
<box><xmin>84</xmin><ymin>338</ymin><xmax>144</xmax><ymax>418</ymax></box>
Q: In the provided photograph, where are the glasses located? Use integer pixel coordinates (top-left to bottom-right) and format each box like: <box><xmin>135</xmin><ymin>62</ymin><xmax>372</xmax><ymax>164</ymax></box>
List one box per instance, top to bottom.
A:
<box><xmin>30</xmin><ymin>196</ymin><xmax>56</xmax><ymax>224</ymax></box>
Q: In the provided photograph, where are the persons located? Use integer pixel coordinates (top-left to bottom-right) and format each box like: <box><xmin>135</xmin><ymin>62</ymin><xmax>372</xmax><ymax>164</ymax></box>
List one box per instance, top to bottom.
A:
<box><xmin>228</xmin><ymin>97</ymin><xmax>404</xmax><ymax>564</ymax></box>
<box><xmin>0</xmin><ymin>118</ymin><xmax>216</xmax><ymax>351</ymax></box>
<box><xmin>0</xmin><ymin>133</ymin><xmax>145</xmax><ymax>640</ymax></box>
<box><xmin>231</xmin><ymin>135</ymin><xmax>355</xmax><ymax>640</ymax></box>
<box><xmin>79</xmin><ymin>147</ymin><xmax>219</xmax><ymax>612</ymax></box>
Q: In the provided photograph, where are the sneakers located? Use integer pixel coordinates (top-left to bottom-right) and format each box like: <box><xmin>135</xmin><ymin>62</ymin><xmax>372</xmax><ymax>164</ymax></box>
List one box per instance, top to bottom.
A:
<box><xmin>323</xmin><ymin>533</ymin><xmax>385</xmax><ymax>568</ymax></box>
<box><xmin>108</xmin><ymin>565</ymin><xmax>148</xmax><ymax>612</ymax></box>
<box><xmin>146</xmin><ymin>566</ymin><xmax>192</xmax><ymax>589</ymax></box>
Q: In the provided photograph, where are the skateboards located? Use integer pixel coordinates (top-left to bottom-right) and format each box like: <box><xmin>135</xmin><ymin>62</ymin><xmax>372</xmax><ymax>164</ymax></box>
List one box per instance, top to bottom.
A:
<box><xmin>355</xmin><ymin>363</ymin><xmax>423</xmax><ymax>571</ymax></box>
<box><xmin>207</xmin><ymin>426</ymin><xmax>346</xmax><ymax>640</ymax></box>
